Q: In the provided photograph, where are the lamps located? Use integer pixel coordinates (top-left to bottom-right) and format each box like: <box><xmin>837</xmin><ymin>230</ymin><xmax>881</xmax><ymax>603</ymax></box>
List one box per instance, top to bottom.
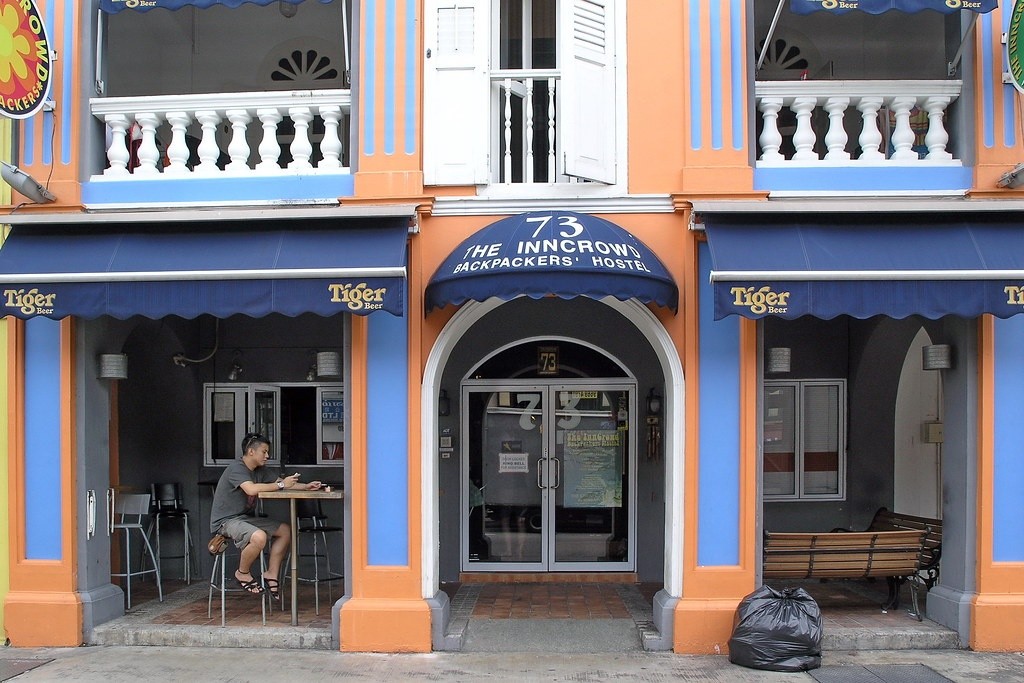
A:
<box><xmin>0</xmin><ymin>160</ymin><xmax>56</xmax><ymax>204</ymax></box>
<box><xmin>996</xmin><ymin>162</ymin><xmax>1024</xmax><ymax>188</ymax></box>
<box><xmin>923</xmin><ymin>344</ymin><xmax>953</xmax><ymax>368</ymax></box>
<box><xmin>764</xmin><ymin>347</ymin><xmax>792</xmax><ymax>374</ymax></box>
<box><xmin>317</xmin><ymin>351</ymin><xmax>342</xmax><ymax>379</ymax></box>
<box><xmin>96</xmin><ymin>354</ymin><xmax>131</xmax><ymax>381</ymax></box>
<box><xmin>306</xmin><ymin>363</ymin><xmax>318</xmax><ymax>382</ymax></box>
<box><xmin>228</xmin><ymin>364</ymin><xmax>243</xmax><ymax>381</ymax></box>
<box><xmin>645</xmin><ymin>386</ymin><xmax>662</xmax><ymax>415</ymax></box>
<box><xmin>436</xmin><ymin>388</ymin><xmax>450</xmax><ymax>416</ymax></box>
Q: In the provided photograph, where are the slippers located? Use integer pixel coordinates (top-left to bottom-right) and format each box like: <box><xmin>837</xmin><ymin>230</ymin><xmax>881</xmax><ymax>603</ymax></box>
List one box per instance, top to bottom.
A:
<box><xmin>234</xmin><ymin>575</ymin><xmax>267</xmax><ymax>596</ymax></box>
<box><xmin>262</xmin><ymin>576</ymin><xmax>280</xmax><ymax>602</ymax></box>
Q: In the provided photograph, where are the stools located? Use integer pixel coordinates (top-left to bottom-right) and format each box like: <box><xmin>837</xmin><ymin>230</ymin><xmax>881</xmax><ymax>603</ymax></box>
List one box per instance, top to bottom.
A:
<box><xmin>141</xmin><ymin>478</ymin><xmax>198</xmax><ymax>588</ymax></box>
<box><xmin>201</xmin><ymin>478</ymin><xmax>273</xmax><ymax>628</ymax></box>
<box><xmin>109</xmin><ymin>493</ymin><xmax>164</xmax><ymax>610</ymax></box>
<box><xmin>280</xmin><ymin>496</ymin><xmax>345</xmax><ymax>616</ymax></box>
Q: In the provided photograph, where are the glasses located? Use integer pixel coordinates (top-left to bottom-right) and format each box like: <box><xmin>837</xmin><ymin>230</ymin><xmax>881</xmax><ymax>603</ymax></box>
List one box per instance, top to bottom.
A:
<box><xmin>245</xmin><ymin>432</ymin><xmax>261</xmax><ymax>449</ymax></box>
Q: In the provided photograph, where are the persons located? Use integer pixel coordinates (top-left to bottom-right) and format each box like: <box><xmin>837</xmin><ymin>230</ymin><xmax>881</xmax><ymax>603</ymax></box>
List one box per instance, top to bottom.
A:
<box><xmin>210</xmin><ymin>433</ymin><xmax>321</xmax><ymax>603</ymax></box>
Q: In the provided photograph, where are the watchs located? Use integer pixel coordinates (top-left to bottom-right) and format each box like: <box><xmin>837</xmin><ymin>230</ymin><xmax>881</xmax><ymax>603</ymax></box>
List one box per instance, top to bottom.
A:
<box><xmin>278</xmin><ymin>481</ymin><xmax>285</xmax><ymax>491</ymax></box>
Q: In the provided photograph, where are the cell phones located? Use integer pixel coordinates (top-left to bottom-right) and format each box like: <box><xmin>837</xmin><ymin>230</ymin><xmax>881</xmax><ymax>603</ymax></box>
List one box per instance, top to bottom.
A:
<box><xmin>295</xmin><ymin>472</ymin><xmax>298</xmax><ymax>476</ymax></box>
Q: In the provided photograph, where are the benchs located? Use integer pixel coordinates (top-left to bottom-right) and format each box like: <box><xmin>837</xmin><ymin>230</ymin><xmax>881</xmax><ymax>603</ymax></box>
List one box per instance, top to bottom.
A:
<box><xmin>830</xmin><ymin>505</ymin><xmax>943</xmax><ymax>610</ymax></box>
<box><xmin>763</xmin><ymin>526</ymin><xmax>932</xmax><ymax>620</ymax></box>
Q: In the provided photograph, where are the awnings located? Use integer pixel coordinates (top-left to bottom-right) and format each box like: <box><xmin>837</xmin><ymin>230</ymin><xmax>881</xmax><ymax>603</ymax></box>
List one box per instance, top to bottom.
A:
<box><xmin>0</xmin><ymin>216</ymin><xmax>410</xmax><ymax>318</ymax></box>
<box><xmin>704</xmin><ymin>218</ymin><xmax>1024</xmax><ymax>323</ymax></box>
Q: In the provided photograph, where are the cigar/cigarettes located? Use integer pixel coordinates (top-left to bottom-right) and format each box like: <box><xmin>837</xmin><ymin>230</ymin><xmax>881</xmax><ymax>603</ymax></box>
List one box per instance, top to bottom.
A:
<box><xmin>319</xmin><ymin>484</ymin><xmax>327</xmax><ymax>486</ymax></box>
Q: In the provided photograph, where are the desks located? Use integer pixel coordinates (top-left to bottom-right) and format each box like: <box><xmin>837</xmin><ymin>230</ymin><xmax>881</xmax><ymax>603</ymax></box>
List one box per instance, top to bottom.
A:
<box><xmin>258</xmin><ymin>488</ymin><xmax>344</xmax><ymax>626</ymax></box>
